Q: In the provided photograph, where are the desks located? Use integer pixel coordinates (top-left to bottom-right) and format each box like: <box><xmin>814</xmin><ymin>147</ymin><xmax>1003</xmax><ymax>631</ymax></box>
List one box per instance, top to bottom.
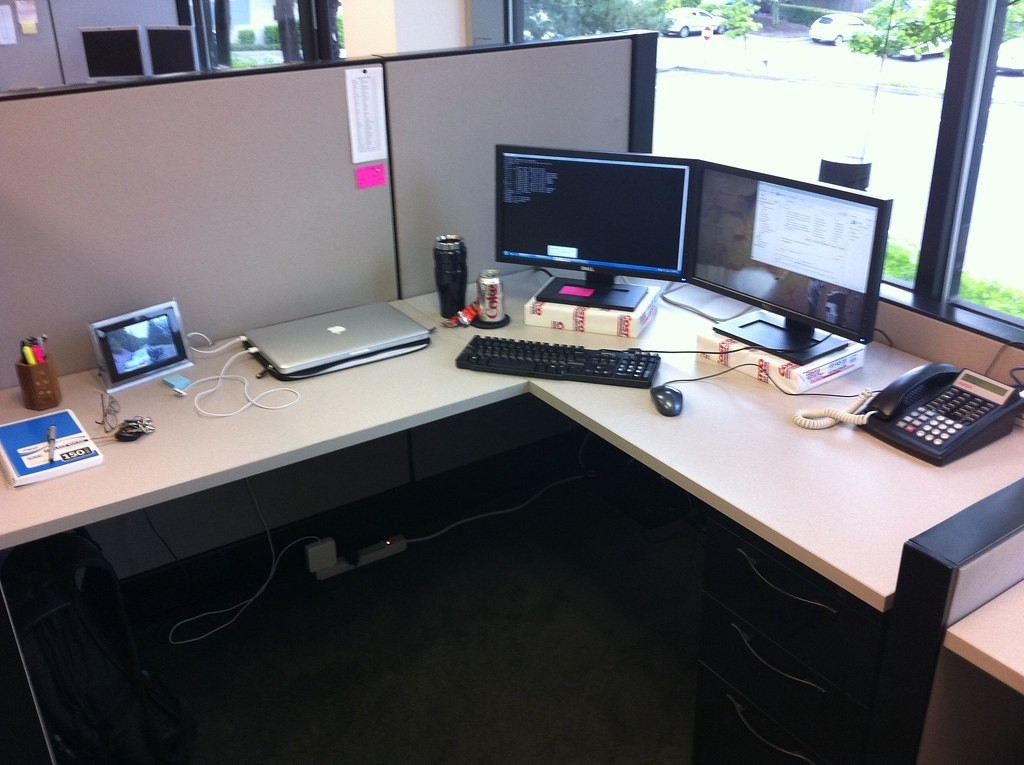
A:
<box><xmin>1</xmin><ymin>266</ymin><xmax>1024</xmax><ymax>765</ymax></box>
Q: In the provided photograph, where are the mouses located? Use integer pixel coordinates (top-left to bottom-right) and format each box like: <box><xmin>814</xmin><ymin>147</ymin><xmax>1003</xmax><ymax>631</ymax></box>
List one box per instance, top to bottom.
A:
<box><xmin>650</xmin><ymin>385</ymin><xmax>683</xmax><ymax>416</ymax></box>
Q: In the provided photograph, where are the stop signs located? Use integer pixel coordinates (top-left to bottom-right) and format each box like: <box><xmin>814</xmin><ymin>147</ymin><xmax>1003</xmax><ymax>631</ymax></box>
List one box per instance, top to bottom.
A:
<box><xmin>703</xmin><ymin>25</ymin><xmax>711</xmax><ymax>40</ymax></box>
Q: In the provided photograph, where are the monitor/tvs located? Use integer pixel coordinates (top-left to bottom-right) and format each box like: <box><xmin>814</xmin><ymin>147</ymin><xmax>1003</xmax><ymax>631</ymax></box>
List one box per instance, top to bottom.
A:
<box><xmin>78</xmin><ymin>24</ymin><xmax>201</xmax><ymax>83</ymax></box>
<box><xmin>494</xmin><ymin>144</ymin><xmax>697</xmax><ymax>312</ymax></box>
<box><xmin>684</xmin><ymin>159</ymin><xmax>893</xmax><ymax>366</ymax></box>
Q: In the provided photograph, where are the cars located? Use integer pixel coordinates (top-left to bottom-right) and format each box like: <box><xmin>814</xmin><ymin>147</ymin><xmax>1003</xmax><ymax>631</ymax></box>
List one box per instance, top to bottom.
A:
<box><xmin>660</xmin><ymin>6</ymin><xmax>730</xmax><ymax>38</ymax></box>
<box><xmin>810</xmin><ymin>13</ymin><xmax>877</xmax><ymax>47</ymax></box>
<box><xmin>894</xmin><ymin>35</ymin><xmax>954</xmax><ymax>62</ymax></box>
<box><xmin>996</xmin><ymin>37</ymin><xmax>1024</xmax><ymax>74</ymax></box>
<box><xmin>124</xmin><ymin>346</ymin><xmax>158</xmax><ymax>370</ymax></box>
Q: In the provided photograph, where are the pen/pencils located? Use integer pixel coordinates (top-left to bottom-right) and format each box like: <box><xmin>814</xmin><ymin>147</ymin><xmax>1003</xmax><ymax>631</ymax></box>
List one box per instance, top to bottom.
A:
<box><xmin>20</xmin><ymin>334</ymin><xmax>48</xmax><ymax>364</ymax></box>
<box><xmin>47</xmin><ymin>424</ymin><xmax>57</xmax><ymax>463</ymax></box>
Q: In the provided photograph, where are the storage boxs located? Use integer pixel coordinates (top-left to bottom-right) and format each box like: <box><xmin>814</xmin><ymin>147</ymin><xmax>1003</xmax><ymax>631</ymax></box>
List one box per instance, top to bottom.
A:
<box><xmin>524</xmin><ymin>276</ymin><xmax>662</xmax><ymax>337</ymax></box>
<box><xmin>697</xmin><ymin>309</ymin><xmax>866</xmax><ymax>395</ymax></box>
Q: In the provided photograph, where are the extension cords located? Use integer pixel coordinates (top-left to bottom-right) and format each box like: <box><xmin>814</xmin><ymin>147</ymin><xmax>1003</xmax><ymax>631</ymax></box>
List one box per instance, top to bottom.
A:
<box><xmin>315</xmin><ymin>535</ymin><xmax>407</xmax><ymax>579</ymax></box>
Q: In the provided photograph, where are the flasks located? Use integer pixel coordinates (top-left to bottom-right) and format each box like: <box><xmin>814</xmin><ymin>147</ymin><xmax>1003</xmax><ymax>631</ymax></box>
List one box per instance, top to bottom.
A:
<box><xmin>432</xmin><ymin>235</ymin><xmax>468</xmax><ymax>319</ymax></box>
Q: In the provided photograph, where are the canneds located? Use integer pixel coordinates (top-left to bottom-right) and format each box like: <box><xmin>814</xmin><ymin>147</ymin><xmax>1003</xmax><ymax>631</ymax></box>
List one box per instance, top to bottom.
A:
<box><xmin>476</xmin><ymin>269</ymin><xmax>504</xmax><ymax>322</ymax></box>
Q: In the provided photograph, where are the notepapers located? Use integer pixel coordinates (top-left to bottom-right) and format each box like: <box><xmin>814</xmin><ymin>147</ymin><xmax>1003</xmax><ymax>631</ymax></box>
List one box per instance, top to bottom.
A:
<box><xmin>161</xmin><ymin>374</ymin><xmax>190</xmax><ymax>388</ymax></box>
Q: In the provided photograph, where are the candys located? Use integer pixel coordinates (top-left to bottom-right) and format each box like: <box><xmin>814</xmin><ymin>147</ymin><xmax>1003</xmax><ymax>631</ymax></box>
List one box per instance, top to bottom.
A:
<box><xmin>442</xmin><ymin>300</ymin><xmax>478</xmax><ymax>327</ymax></box>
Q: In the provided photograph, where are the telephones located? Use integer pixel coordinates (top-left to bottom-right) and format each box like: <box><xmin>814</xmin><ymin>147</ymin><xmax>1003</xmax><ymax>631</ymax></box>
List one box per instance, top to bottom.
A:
<box><xmin>853</xmin><ymin>361</ymin><xmax>1024</xmax><ymax>467</ymax></box>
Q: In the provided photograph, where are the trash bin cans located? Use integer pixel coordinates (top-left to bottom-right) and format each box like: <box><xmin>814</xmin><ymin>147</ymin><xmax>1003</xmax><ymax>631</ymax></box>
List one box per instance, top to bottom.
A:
<box><xmin>819</xmin><ymin>155</ymin><xmax>871</xmax><ymax>191</ymax></box>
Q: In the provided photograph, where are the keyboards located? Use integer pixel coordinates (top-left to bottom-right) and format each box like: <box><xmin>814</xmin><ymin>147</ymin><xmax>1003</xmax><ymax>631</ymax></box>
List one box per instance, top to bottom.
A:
<box><xmin>456</xmin><ymin>334</ymin><xmax>661</xmax><ymax>389</ymax></box>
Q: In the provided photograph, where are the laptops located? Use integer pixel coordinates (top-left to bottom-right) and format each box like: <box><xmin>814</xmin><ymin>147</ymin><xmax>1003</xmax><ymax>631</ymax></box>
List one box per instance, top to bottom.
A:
<box><xmin>243</xmin><ymin>301</ymin><xmax>429</xmax><ymax>382</ymax></box>
